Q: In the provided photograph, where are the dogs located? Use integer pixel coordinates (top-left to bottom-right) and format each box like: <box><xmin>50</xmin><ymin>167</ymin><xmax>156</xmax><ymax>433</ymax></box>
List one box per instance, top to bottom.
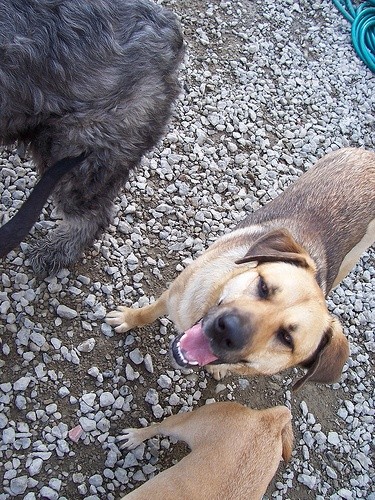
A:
<box><xmin>0</xmin><ymin>0</ymin><xmax>185</xmax><ymax>282</ymax></box>
<box><xmin>102</xmin><ymin>147</ymin><xmax>375</xmax><ymax>393</ymax></box>
<box><xmin>0</xmin><ymin>148</ymin><xmax>93</xmax><ymax>259</ymax></box>
<box><xmin>114</xmin><ymin>402</ymin><xmax>295</xmax><ymax>500</ymax></box>
